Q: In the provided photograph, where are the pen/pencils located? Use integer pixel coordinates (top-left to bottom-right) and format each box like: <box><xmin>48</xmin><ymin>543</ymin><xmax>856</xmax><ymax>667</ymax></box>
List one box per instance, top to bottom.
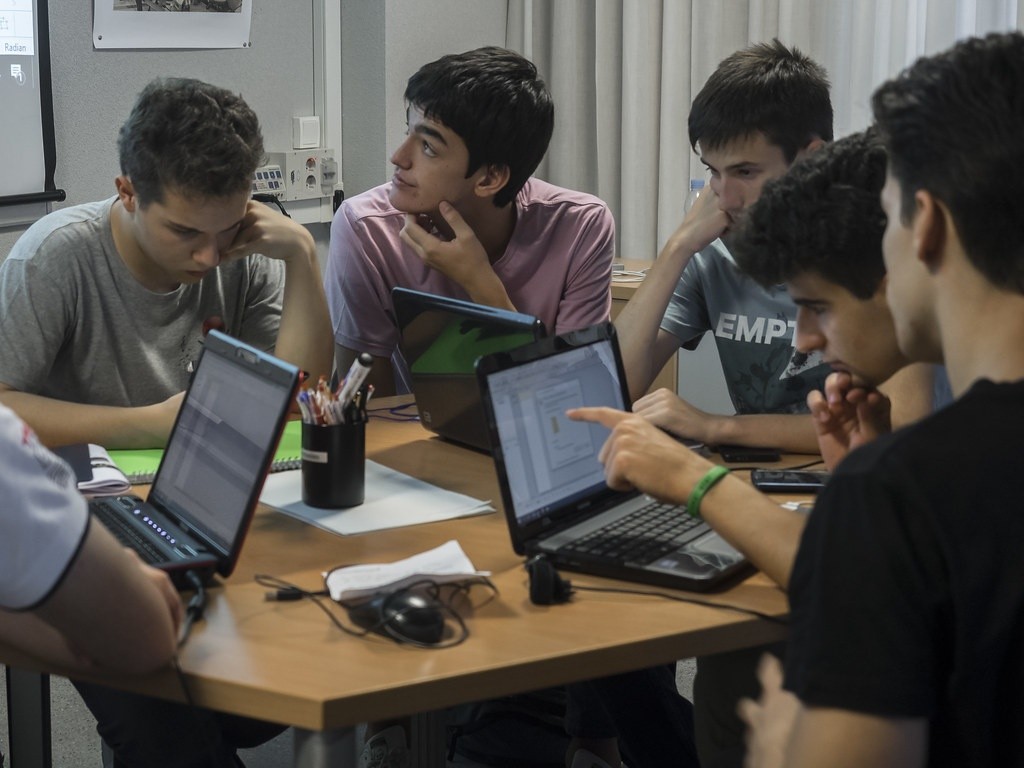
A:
<box><xmin>297</xmin><ymin>368</ymin><xmax>375</xmax><ymax>426</ymax></box>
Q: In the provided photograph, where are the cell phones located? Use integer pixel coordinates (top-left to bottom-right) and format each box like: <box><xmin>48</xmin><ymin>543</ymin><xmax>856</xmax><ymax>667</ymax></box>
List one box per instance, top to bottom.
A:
<box><xmin>717</xmin><ymin>444</ymin><xmax>781</xmax><ymax>462</ymax></box>
<box><xmin>750</xmin><ymin>469</ymin><xmax>830</xmax><ymax>492</ymax></box>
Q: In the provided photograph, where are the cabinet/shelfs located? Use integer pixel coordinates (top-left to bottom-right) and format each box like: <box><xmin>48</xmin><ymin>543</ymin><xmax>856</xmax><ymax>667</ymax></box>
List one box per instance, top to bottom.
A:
<box><xmin>609</xmin><ymin>257</ymin><xmax>678</xmax><ymax>398</ymax></box>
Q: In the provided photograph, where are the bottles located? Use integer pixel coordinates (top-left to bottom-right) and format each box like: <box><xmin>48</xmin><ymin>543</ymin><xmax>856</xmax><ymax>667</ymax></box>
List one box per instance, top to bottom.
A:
<box><xmin>685</xmin><ymin>179</ymin><xmax>705</xmax><ymax>215</ymax></box>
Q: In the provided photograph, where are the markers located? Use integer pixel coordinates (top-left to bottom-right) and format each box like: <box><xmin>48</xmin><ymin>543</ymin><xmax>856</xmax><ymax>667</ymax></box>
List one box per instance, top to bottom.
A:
<box><xmin>338</xmin><ymin>352</ymin><xmax>374</xmax><ymax>409</ymax></box>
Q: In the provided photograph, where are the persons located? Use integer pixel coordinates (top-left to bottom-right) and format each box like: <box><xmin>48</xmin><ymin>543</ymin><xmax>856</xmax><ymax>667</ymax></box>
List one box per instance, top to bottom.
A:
<box><xmin>741</xmin><ymin>34</ymin><xmax>1024</xmax><ymax>768</ymax></box>
<box><xmin>612</xmin><ymin>40</ymin><xmax>902</xmax><ymax>768</ymax></box>
<box><xmin>322</xmin><ymin>45</ymin><xmax>629</xmax><ymax>768</ymax></box>
<box><xmin>0</xmin><ymin>77</ymin><xmax>335</xmax><ymax>768</ymax></box>
<box><xmin>566</xmin><ymin>129</ymin><xmax>967</xmax><ymax>768</ymax></box>
<box><xmin>0</xmin><ymin>401</ymin><xmax>182</xmax><ymax>768</ymax></box>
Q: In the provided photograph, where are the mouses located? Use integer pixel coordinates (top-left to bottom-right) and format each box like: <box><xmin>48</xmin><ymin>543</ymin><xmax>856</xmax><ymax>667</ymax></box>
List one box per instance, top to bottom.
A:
<box><xmin>349</xmin><ymin>588</ymin><xmax>447</xmax><ymax>645</ymax></box>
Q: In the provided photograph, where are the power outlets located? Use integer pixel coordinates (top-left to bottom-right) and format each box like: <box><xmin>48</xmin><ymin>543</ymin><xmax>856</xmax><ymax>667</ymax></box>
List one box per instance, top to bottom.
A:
<box><xmin>321</xmin><ymin>181</ymin><xmax>345</xmax><ymax>225</ymax></box>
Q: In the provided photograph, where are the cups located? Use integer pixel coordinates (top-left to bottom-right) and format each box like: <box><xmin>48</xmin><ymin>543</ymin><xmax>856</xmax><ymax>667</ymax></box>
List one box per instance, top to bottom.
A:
<box><xmin>301</xmin><ymin>418</ymin><xmax>366</xmax><ymax>510</ymax></box>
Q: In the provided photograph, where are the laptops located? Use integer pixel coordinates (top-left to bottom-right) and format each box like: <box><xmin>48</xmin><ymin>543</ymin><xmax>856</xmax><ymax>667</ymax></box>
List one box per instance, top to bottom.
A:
<box><xmin>390</xmin><ymin>286</ymin><xmax>812</xmax><ymax>595</ymax></box>
<box><xmin>87</xmin><ymin>330</ymin><xmax>303</xmax><ymax>589</ymax></box>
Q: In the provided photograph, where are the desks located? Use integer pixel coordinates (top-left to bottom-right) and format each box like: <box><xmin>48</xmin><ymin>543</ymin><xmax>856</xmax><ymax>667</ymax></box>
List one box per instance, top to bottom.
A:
<box><xmin>0</xmin><ymin>390</ymin><xmax>829</xmax><ymax>768</ymax></box>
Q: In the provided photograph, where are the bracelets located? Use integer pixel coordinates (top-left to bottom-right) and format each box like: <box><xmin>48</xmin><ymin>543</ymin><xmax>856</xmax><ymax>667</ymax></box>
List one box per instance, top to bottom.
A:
<box><xmin>688</xmin><ymin>465</ymin><xmax>729</xmax><ymax>517</ymax></box>
